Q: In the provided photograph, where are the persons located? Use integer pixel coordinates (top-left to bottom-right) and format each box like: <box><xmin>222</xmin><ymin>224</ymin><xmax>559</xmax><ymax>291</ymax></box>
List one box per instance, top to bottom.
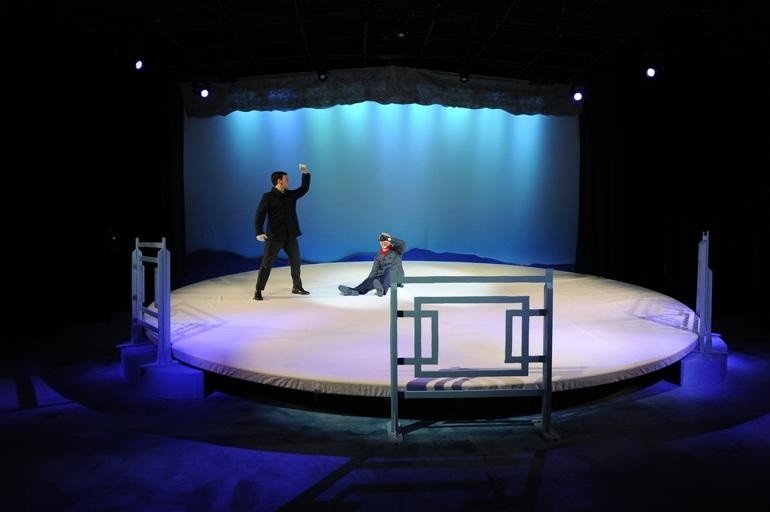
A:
<box><xmin>253</xmin><ymin>164</ymin><xmax>311</xmax><ymax>301</ymax></box>
<box><xmin>337</xmin><ymin>231</ymin><xmax>407</xmax><ymax>297</ymax></box>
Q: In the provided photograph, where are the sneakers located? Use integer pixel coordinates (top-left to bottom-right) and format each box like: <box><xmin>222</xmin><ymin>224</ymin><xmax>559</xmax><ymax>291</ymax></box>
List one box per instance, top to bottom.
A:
<box><xmin>373</xmin><ymin>279</ymin><xmax>384</xmax><ymax>297</ymax></box>
<box><xmin>339</xmin><ymin>286</ymin><xmax>359</xmax><ymax>296</ymax></box>
<box><xmin>292</xmin><ymin>288</ymin><xmax>309</xmax><ymax>295</ymax></box>
<box><xmin>255</xmin><ymin>291</ymin><xmax>262</xmax><ymax>301</ymax></box>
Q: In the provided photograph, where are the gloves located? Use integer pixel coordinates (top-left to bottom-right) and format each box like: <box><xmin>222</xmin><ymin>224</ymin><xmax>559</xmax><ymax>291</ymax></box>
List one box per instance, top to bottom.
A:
<box><xmin>379</xmin><ymin>235</ymin><xmax>391</xmax><ymax>241</ymax></box>
